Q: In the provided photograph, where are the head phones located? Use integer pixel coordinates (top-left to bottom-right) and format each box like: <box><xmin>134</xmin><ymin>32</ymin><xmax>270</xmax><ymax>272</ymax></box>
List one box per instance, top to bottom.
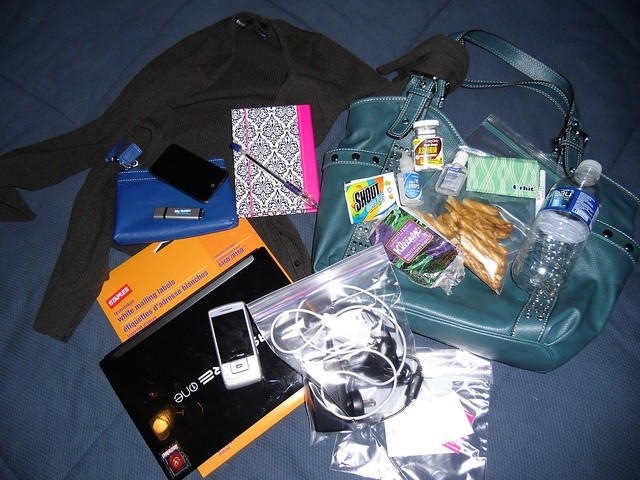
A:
<box><xmin>346</xmin><ymin>335</ymin><xmax>397</xmax><ymax>417</ymax></box>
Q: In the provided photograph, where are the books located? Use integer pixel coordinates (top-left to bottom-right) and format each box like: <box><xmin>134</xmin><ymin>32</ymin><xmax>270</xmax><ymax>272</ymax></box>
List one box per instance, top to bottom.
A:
<box><xmin>230</xmin><ymin>103</ymin><xmax>320</xmax><ymax>218</ymax></box>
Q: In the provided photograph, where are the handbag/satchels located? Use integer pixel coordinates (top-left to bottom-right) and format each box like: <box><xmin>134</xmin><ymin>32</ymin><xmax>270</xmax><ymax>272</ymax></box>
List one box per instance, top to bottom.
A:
<box><xmin>312</xmin><ymin>29</ymin><xmax>639</xmax><ymax>374</ymax></box>
<box><xmin>101</xmin><ymin>140</ymin><xmax>239</xmax><ymax>246</ymax></box>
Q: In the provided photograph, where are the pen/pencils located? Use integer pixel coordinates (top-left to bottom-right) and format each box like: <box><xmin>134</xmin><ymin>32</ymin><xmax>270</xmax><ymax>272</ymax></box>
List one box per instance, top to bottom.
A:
<box><xmin>231</xmin><ymin>143</ymin><xmax>319</xmax><ymax>209</ymax></box>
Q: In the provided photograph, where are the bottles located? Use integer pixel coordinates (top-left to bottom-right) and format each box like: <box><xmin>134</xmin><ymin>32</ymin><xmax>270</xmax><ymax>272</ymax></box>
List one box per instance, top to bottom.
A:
<box><xmin>412</xmin><ymin>120</ymin><xmax>444</xmax><ymax>172</ymax></box>
<box><xmin>434</xmin><ymin>150</ymin><xmax>470</xmax><ymax>197</ymax></box>
<box><xmin>397</xmin><ymin>151</ymin><xmax>424</xmax><ymax>208</ymax></box>
<box><xmin>512</xmin><ymin>159</ymin><xmax>602</xmax><ymax>298</ymax></box>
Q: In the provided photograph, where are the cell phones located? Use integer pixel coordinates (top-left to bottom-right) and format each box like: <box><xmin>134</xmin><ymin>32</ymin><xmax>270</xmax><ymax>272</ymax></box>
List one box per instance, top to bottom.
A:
<box><xmin>148</xmin><ymin>143</ymin><xmax>230</xmax><ymax>205</ymax></box>
<box><xmin>207</xmin><ymin>302</ymin><xmax>262</xmax><ymax>391</ymax></box>
